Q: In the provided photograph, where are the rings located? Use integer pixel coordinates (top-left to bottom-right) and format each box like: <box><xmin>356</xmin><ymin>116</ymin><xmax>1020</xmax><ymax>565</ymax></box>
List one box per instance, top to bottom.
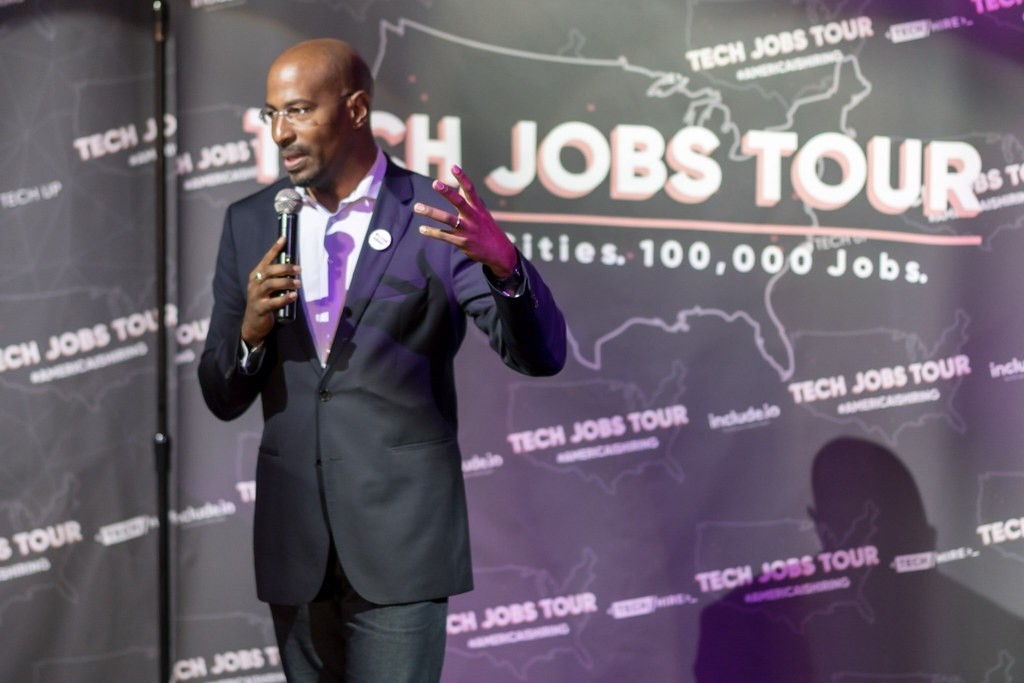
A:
<box><xmin>454</xmin><ymin>217</ymin><xmax>462</xmax><ymax>228</ymax></box>
<box><xmin>254</xmin><ymin>272</ymin><xmax>263</xmax><ymax>285</ymax></box>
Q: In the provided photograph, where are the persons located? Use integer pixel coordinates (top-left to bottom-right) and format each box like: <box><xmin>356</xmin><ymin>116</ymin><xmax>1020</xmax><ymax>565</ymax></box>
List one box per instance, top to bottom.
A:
<box><xmin>198</xmin><ymin>36</ymin><xmax>568</xmax><ymax>683</ymax></box>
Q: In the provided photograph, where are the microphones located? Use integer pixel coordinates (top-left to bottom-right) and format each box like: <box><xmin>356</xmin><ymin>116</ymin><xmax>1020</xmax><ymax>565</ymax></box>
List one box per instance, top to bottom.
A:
<box><xmin>274</xmin><ymin>189</ymin><xmax>305</xmax><ymax>322</ymax></box>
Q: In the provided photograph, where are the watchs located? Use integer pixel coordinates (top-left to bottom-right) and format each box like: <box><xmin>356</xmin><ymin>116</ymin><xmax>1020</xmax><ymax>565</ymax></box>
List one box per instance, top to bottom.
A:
<box><xmin>494</xmin><ymin>261</ymin><xmax>522</xmax><ymax>289</ymax></box>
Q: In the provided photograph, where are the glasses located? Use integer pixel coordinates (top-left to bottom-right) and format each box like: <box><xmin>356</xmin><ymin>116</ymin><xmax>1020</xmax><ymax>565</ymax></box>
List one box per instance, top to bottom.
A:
<box><xmin>260</xmin><ymin>94</ymin><xmax>353</xmax><ymax>125</ymax></box>
